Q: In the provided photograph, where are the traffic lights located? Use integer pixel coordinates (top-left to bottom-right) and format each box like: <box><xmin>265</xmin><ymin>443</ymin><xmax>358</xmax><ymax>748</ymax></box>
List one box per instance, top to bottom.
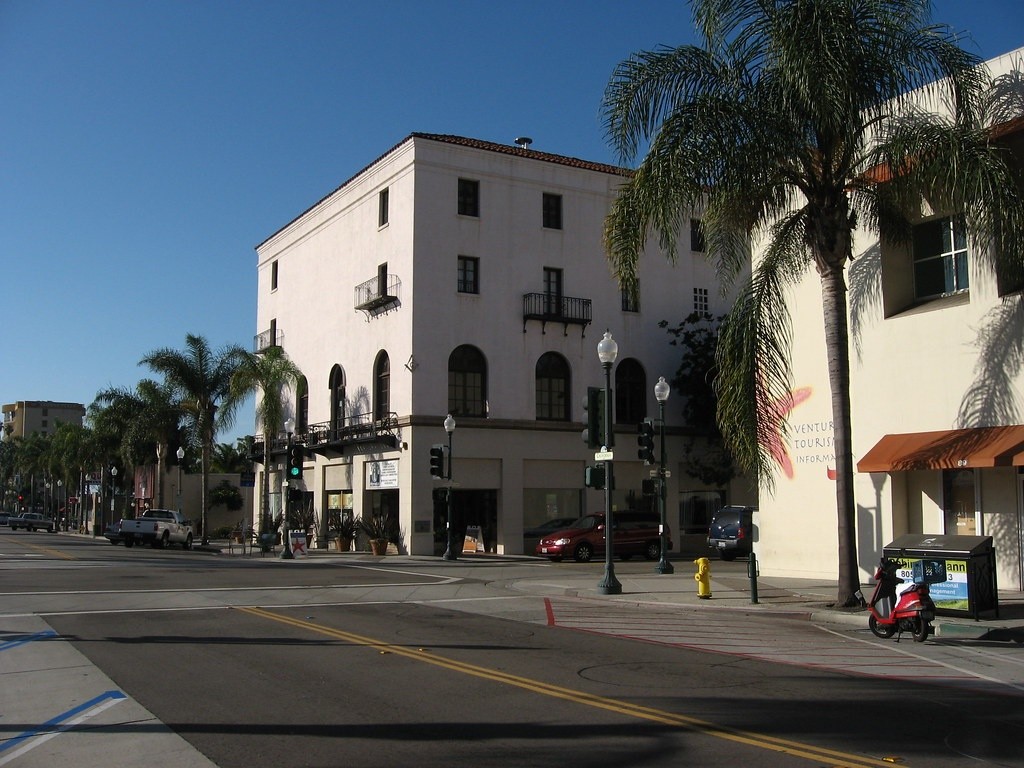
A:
<box><xmin>582</xmin><ymin>387</ymin><xmax>602</xmax><ymax>451</ymax></box>
<box><xmin>638</xmin><ymin>418</ymin><xmax>654</xmax><ymax>466</ymax></box>
<box><xmin>286</xmin><ymin>445</ymin><xmax>304</xmax><ymax>480</ymax></box>
<box><xmin>429</xmin><ymin>445</ymin><xmax>442</xmax><ymax>480</ymax></box>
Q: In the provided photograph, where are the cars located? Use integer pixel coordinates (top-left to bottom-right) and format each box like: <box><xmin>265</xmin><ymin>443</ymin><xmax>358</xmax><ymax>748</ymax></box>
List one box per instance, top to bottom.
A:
<box><xmin>0</xmin><ymin>511</ymin><xmax>27</xmax><ymax>526</ymax></box>
<box><xmin>105</xmin><ymin>521</ymin><xmax>138</xmax><ymax>546</ymax></box>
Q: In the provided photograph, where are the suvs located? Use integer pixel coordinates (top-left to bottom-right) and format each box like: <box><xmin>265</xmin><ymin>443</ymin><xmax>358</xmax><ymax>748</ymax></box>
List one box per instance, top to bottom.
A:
<box><xmin>708</xmin><ymin>505</ymin><xmax>758</xmax><ymax>559</ymax></box>
<box><xmin>537</xmin><ymin>511</ymin><xmax>673</xmax><ymax>561</ymax></box>
<box><xmin>524</xmin><ymin>518</ymin><xmax>575</xmax><ymax>538</ymax></box>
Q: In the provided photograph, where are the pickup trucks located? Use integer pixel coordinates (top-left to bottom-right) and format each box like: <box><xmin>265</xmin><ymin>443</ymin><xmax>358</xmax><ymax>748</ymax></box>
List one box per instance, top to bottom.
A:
<box><xmin>120</xmin><ymin>508</ymin><xmax>196</xmax><ymax>550</ymax></box>
<box><xmin>7</xmin><ymin>513</ymin><xmax>52</xmax><ymax>533</ymax></box>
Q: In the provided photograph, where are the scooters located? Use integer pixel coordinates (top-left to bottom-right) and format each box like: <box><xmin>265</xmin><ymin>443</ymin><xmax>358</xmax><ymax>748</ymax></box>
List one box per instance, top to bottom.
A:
<box><xmin>869</xmin><ymin>549</ymin><xmax>939</xmax><ymax>642</ymax></box>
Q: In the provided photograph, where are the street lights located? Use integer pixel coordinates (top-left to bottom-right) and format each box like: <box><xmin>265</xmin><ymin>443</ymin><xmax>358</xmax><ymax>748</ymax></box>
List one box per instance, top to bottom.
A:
<box><xmin>597</xmin><ymin>329</ymin><xmax>624</xmax><ymax>595</ymax></box>
<box><xmin>653</xmin><ymin>376</ymin><xmax>675</xmax><ymax>574</ymax></box>
<box><xmin>444</xmin><ymin>414</ymin><xmax>459</xmax><ymax>559</ymax></box>
<box><xmin>279</xmin><ymin>418</ymin><xmax>295</xmax><ymax>560</ymax></box>
<box><xmin>175</xmin><ymin>448</ymin><xmax>185</xmax><ymax>512</ymax></box>
<box><xmin>110</xmin><ymin>467</ymin><xmax>117</xmax><ymax>524</ymax></box>
<box><xmin>45</xmin><ymin>473</ymin><xmax>91</xmax><ymax>534</ymax></box>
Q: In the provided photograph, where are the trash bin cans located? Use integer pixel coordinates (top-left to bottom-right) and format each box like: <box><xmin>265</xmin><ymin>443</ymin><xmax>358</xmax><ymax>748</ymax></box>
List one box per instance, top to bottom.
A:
<box><xmin>71</xmin><ymin>519</ymin><xmax>78</xmax><ymax>530</ymax></box>
<box><xmin>882</xmin><ymin>534</ymin><xmax>999</xmax><ymax>622</ymax></box>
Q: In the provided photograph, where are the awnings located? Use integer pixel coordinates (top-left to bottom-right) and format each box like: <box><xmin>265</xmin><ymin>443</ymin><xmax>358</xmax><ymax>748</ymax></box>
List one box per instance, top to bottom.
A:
<box><xmin>844</xmin><ymin>115</ymin><xmax>1024</xmax><ymax>192</ymax></box>
<box><xmin>857</xmin><ymin>425</ymin><xmax>1024</xmax><ymax>473</ymax></box>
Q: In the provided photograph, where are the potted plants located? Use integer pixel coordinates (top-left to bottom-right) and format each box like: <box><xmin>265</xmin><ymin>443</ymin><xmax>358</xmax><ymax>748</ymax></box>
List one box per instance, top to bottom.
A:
<box><xmin>288</xmin><ymin>507</ymin><xmax>314</xmax><ymax>548</ymax></box>
<box><xmin>260</xmin><ymin>512</ymin><xmax>284</xmax><ymax>544</ymax></box>
<box><xmin>329</xmin><ymin>513</ymin><xmax>359</xmax><ymax>552</ymax></box>
<box><xmin>356</xmin><ymin>513</ymin><xmax>394</xmax><ymax>555</ymax></box>
<box><xmin>234</xmin><ymin>520</ymin><xmax>243</xmax><ymax>543</ymax></box>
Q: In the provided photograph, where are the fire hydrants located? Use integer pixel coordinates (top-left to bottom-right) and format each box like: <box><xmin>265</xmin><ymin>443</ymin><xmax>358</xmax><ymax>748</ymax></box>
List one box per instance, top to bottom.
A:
<box><xmin>692</xmin><ymin>557</ymin><xmax>713</xmax><ymax>601</ymax></box>
